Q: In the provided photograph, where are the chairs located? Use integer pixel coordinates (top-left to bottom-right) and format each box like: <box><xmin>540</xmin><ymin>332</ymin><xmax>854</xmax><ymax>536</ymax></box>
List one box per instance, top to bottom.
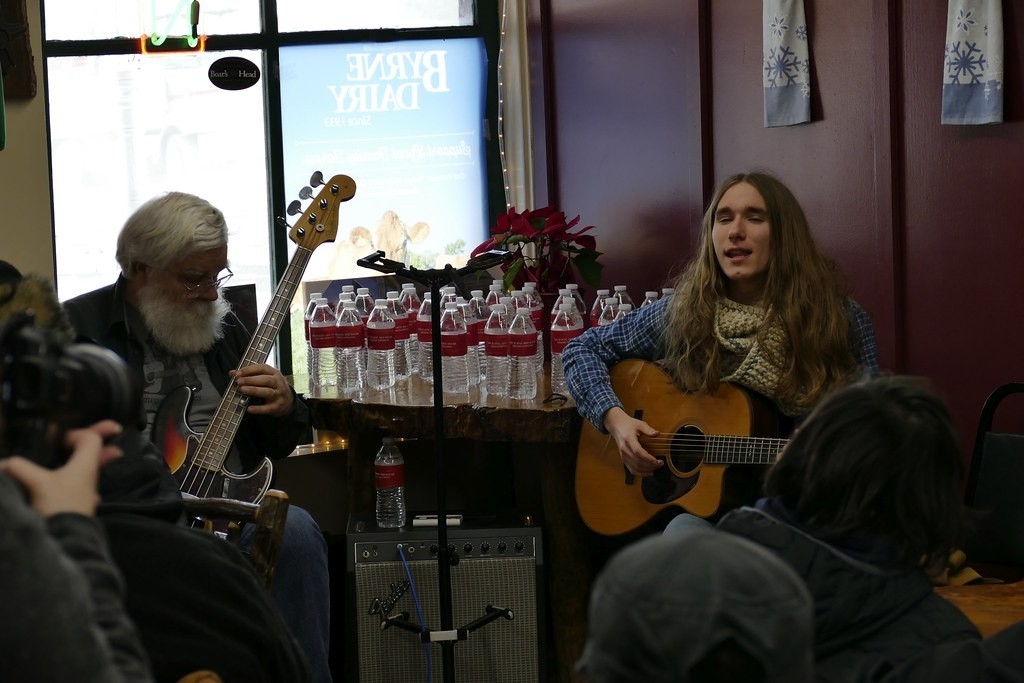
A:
<box><xmin>954</xmin><ymin>382</ymin><xmax>1024</xmax><ymax>582</ymax></box>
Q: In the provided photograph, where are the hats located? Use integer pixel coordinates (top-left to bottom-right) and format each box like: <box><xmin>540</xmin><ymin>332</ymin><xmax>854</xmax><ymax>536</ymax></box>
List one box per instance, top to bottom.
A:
<box><xmin>573</xmin><ymin>529</ymin><xmax>813</xmax><ymax>683</ymax></box>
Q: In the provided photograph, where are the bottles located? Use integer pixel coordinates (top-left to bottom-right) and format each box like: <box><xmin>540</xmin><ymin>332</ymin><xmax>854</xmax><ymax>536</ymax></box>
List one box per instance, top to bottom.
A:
<box><xmin>301</xmin><ymin>279</ymin><xmax>677</xmax><ymax>400</ymax></box>
<box><xmin>374</xmin><ymin>436</ymin><xmax>406</xmax><ymax>527</ymax></box>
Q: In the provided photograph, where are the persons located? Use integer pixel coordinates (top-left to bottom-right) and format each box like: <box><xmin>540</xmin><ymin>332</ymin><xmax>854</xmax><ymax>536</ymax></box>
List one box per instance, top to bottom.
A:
<box><xmin>562</xmin><ymin>172</ymin><xmax>887</xmax><ymax>519</ymax></box>
<box><xmin>58</xmin><ymin>185</ymin><xmax>337</xmax><ymax>683</ymax></box>
<box><xmin>1</xmin><ymin>258</ymin><xmax>315</xmax><ymax>683</ymax></box>
<box><xmin>717</xmin><ymin>367</ymin><xmax>986</xmax><ymax>683</ymax></box>
<box><xmin>571</xmin><ymin>530</ymin><xmax>819</xmax><ymax>683</ymax></box>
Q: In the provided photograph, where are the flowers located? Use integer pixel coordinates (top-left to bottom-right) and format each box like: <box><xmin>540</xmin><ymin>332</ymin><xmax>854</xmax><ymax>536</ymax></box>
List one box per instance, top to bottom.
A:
<box><xmin>469</xmin><ymin>206</ymin><xmax>606</xmax><ymax>304</ymax></box>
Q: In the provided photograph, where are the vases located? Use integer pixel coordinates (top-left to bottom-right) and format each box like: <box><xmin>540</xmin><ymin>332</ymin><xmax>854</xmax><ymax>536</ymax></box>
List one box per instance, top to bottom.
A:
<box><xmin>539</xmin><ymin>294</ymin><xmax>559</xmax><ymax>361</ymax></box>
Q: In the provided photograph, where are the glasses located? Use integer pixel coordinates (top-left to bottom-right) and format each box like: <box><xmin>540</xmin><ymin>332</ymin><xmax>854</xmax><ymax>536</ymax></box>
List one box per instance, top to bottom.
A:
<box><xmin>167</xmin><ymin>267</ymin><xmax>233</xmax><ymax>292</ymax></box>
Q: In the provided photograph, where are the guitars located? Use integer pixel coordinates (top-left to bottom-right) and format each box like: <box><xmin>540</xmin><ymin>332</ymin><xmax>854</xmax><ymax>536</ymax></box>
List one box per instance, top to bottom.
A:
<box><xmin>154</xmin><ymin>170</ymin><xmax>358</xmax><ymax>535</ymax></box>
<box><xmin>574</xmin><ymin>356</ymin><xmax>790</xmax><ymax>538</ymax></box>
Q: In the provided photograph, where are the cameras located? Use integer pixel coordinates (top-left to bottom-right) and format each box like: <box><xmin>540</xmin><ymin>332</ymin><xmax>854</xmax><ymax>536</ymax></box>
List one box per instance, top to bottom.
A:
<box><xmin>0</xmin><ymin>307</ymin><xmax>139</xmax><ymax>467</ymax></box>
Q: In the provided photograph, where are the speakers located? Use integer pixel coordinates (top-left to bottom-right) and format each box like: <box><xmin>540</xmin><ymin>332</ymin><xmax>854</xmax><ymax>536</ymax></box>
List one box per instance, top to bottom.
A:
<box><xmin>346</xmin><ymin>511</ymin><xmax>543</xmax><ymax>683</ymax></box>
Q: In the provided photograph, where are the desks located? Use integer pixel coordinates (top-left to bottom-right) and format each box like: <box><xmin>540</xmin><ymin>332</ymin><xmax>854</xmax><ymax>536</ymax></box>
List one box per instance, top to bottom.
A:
<box><xmin>282</xmin><ymin>373</ymin><xmax>595</xmax><ymax>683</ymax></box>
